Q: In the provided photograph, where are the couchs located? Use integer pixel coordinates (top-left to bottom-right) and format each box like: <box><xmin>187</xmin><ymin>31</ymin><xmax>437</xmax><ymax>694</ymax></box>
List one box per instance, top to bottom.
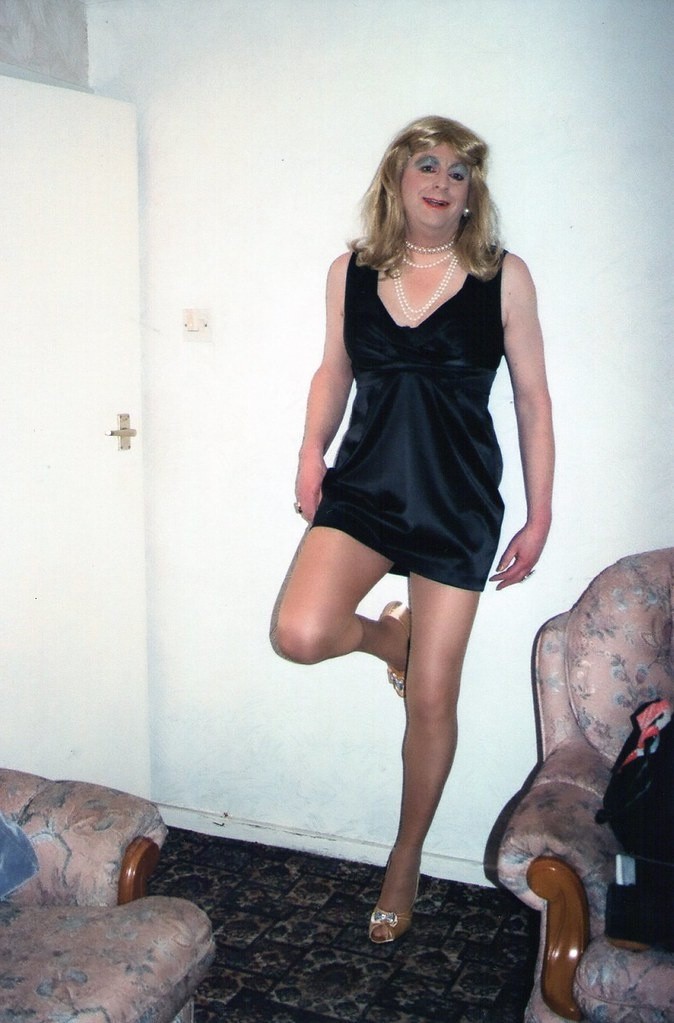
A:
<box><xmin>496</xmin><ymin>547</ymin><xmax>674</xmax><ymax>1023</ymax></box>
<box><xmin>0</xmin><ymin>766</ymin><xmax>216</xmax><ymax>1023</ymax></box>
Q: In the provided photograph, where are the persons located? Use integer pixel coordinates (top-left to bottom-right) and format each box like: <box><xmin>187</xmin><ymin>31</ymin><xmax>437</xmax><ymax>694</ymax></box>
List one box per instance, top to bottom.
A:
<box><xmin>274</xmin><ymin>115</ymin><xmax>555</xmax><ymax>944</ymax></box>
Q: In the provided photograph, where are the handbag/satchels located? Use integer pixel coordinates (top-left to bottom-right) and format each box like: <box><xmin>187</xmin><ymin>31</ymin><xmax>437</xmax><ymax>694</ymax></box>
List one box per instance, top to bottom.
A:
<box><xmin>595</xmin><ymin>697</ymin><xmax>674</xmax><ymax>865</ymax></box>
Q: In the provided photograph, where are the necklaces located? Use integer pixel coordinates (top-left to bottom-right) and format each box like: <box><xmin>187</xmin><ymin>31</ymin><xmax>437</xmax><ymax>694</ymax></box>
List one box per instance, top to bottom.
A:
<box><xmin>392</xmin><ymin>241</ymin><xmax>458</xmax><ymax>322</ymax></box>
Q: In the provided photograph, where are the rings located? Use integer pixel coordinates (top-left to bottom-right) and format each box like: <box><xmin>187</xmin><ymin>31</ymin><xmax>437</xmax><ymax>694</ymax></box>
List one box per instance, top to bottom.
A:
<box><xmin>297</xmin><ymin>502</ymin><xmax>302</xmax><ymax>512</ymax></box>
<box><xmin>521</xmin><ymin>570</ymin><xmax>535</xmax><ymax>582</ymax></box>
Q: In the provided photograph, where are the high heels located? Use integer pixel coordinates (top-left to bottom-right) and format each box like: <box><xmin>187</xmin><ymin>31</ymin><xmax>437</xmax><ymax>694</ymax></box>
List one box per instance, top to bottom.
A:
<box><xmin>378</xmin><ymin>602</ymin><xmax>411</xmax><ymax>697</ymax></box>
<box><xmin>369</xmin><ymin>846</ymin><xmax>421</xmax><ymax>943</ymax></box>
